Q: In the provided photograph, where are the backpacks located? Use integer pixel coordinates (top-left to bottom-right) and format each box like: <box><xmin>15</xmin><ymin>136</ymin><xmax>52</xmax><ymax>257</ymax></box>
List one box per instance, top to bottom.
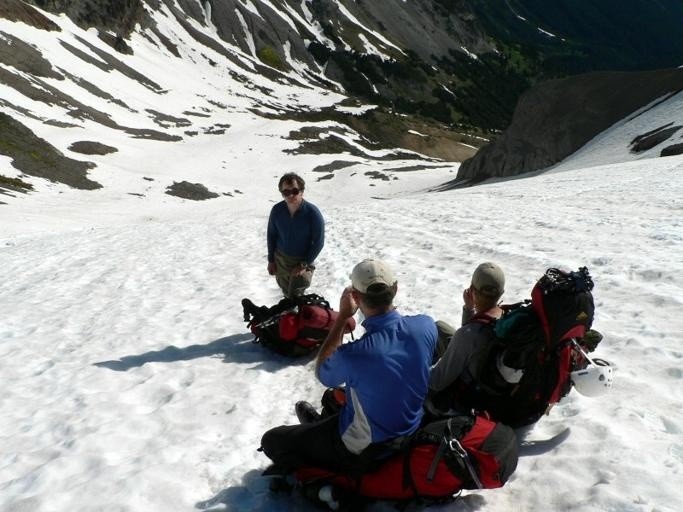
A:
<box><xmin>505</xmin><ymin>268</ymin><xmax>594</xmax><ymax>428</ymax></box>
<box><xmin>240</xmin><ymin>295</ymin><xmax>355</xmax><ymax>359</ymax></box>
<box><xmin>361</xmin><ymin>415</ymin><xmax>518</xmax><ymax>506</ymax></box>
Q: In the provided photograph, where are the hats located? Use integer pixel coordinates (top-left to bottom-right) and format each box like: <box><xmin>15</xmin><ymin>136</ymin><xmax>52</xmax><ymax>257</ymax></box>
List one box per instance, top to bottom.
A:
<box><xmin>349</xmin><ymin>258</ymin><xmax>394</xmax><ymax>294</ymax></box>
<box><xmin>471</xmin><ymin>261</ymin><xmax>505</xmax><ymax>296</ymax></box>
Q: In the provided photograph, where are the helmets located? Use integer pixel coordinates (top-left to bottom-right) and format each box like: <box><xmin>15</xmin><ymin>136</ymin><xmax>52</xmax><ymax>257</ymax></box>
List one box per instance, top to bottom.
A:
<box><xmin>565</xmin><ymin>366</ymin><xmax>619</xmax><ymax>397</ymax></box>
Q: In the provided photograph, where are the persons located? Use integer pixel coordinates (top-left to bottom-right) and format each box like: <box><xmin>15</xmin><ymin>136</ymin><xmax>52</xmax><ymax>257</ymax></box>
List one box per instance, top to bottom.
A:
<box><xmin>265</xmin><ymin>172</ymin><xmax>325</xmax><ymax>301</ymax></box>
<box><xmin>260</xmin><ymin>258</ymin><xmax>441</xmax><ymax>508</ymax></box>
<box><xmin>426</xmin><ymin>261</ymin><xmax>515</xmax><ymax>424</ymax></box>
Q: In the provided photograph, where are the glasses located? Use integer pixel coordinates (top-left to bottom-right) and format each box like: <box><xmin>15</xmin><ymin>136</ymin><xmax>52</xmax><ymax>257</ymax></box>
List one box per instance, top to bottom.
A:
<box><xmin>280</xmin><ymin>188</ymin><xmax>300</xmax><ymax>196</ymax></box>
